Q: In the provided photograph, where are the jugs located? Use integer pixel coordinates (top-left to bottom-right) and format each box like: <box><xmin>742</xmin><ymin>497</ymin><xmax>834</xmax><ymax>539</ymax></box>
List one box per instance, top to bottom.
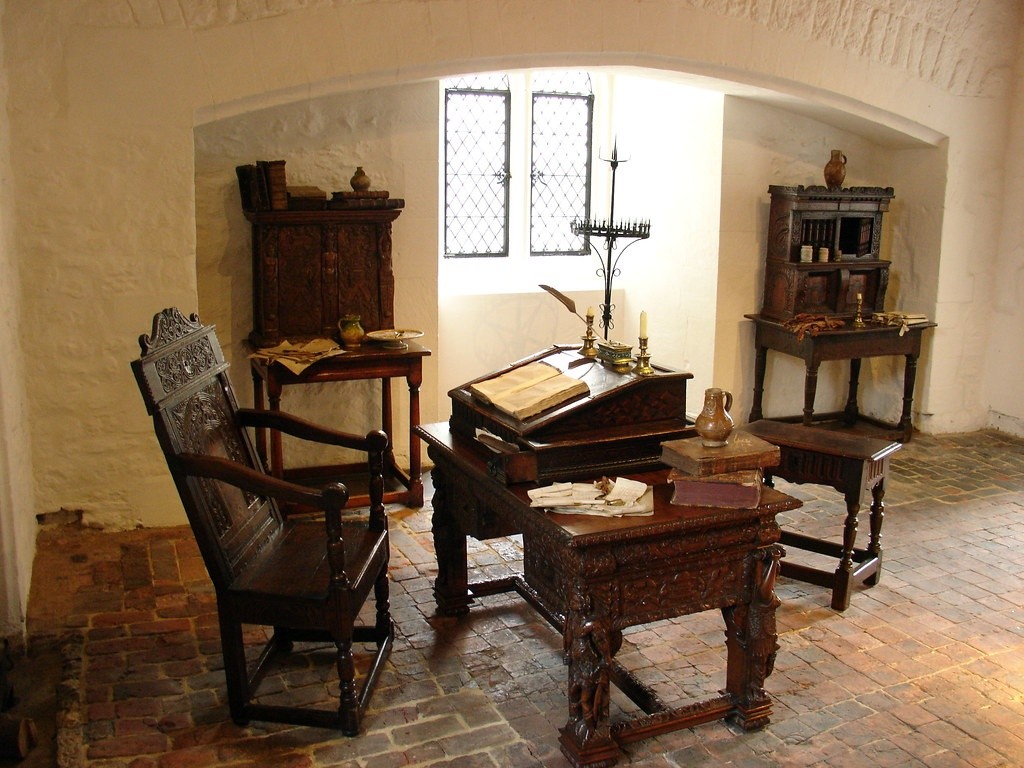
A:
<box><xmin>338</xmin><ymin>313</ymin><xmax>365</xmax><ymax>348</ymax></box>
<box><xmin>695</xmin><ymin>387</ymin><xmax>735</xmax><ymax>447</ymax></box>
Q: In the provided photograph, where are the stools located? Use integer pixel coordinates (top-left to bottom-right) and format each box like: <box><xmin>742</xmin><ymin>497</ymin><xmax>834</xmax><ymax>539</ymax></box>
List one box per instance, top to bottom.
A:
<box><xmin>737</xmin><ymin>419</ymin><xmax>902</xmax><ymax>611</ymax></box>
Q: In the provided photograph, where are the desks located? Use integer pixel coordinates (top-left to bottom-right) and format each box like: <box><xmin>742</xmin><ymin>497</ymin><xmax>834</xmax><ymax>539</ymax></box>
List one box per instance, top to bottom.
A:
<box><xmin>744</xmin><ymin>313</ymin><xmax>938</xmax><ymax>443</ymax></box>
<box><xmin>243</xmin><ymin>339</ymin><xmax>432</xmax><ymax>520</ymax></box>
<box><xmin>413</xmin><ymin>416</ymin><xmax>804</xmax><ymax>768</ymax></box>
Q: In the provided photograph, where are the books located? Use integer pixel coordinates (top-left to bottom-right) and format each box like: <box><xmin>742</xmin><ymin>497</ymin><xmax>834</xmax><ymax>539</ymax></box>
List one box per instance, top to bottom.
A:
<box><xmin>235</xmin><ymin>160</ymin><xmax>405</xmax><ymax>212</ymax></box>
<box><xmin>856</xmin><ymin>218</ymin><xmax>873</xmax><ymax>257</ymax></box>
<box><xmin>800</xmin><ymin>220</ymin><xmax>836</xmax><ymax>259</ymax></box>
<box><xmin>871</xmin><ymin>311</ymin><xmax>929</xmax><ymax>327</ymax></box>
<box><xmin>469</xmin><ymin>360</ymin><xmax>591</xmax><ymax>424</ymax></box>
<box><xmin>658</xmin><ymin>428</ymin><xmax>780</xmax><ymax>509</ymax></box>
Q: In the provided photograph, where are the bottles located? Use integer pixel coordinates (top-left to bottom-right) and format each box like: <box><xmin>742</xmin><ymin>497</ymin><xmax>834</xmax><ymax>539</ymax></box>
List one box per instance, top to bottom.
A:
<box><xmin>824</xmin><ymin>150</ymin><xmax>848</xmax><ymax>187</ymax></box>
<box><xmin>350</xmin><ymin>166</ymin><xmax>372</xmax><ymax>192</ymax></box>
<box><xmin>800</xmin><ymin>245</ymin><xmax>842</xmax><ymax>263</ymax></box>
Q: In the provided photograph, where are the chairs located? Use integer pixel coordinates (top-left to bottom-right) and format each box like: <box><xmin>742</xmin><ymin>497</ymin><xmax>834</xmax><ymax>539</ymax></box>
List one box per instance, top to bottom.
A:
<box><xmin>129</xmin><ymin>306</ymin><xmax>395</xmax><ymax>735</ymax></box>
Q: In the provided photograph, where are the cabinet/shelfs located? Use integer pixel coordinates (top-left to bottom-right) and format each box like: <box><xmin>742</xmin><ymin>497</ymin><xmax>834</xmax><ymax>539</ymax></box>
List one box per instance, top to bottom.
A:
<box><xmin>761</xmin><ymin>185</ymin><xmax>895</xmax><ymax>324</ymax></box>
<box><xmin>243</xmin><ymin>208</ymin><xmax>402</xmax><ymax>347</ymax></box>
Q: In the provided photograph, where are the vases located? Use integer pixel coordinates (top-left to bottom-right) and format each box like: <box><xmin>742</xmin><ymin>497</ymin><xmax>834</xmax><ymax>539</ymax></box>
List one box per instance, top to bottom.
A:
<box><xmin>825</xmin><ymin>150</ymin><xmax>847</xmax><ymax>189</ymax></box>
<box><xmin>695</xmin><ymin>388</ymin><xmax>734</xmax><ymax>448</ymax></box>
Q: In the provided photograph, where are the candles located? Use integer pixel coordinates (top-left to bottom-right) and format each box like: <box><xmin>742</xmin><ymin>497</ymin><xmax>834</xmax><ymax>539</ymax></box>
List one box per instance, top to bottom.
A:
<box><xmin>857</xmin><ymin>293</ymin><xmax>862</xmax><ymax>299</ymax></box>
<box><xmin>588</xmin><ymin>306</ymin><xmax>594</xmax><ymax>316</ymax></box>
<box><xmin>640</xmin><ymin>310</ymin><xmax>648</xmax><ymax>339</ymax></box>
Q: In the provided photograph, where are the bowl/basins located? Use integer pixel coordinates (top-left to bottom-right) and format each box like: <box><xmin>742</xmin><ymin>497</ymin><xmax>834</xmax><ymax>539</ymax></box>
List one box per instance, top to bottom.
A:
<box><xmin>366</xmin><ymin>328</ymin><xmax>424</xmax><ymax>349</ymax></box>
